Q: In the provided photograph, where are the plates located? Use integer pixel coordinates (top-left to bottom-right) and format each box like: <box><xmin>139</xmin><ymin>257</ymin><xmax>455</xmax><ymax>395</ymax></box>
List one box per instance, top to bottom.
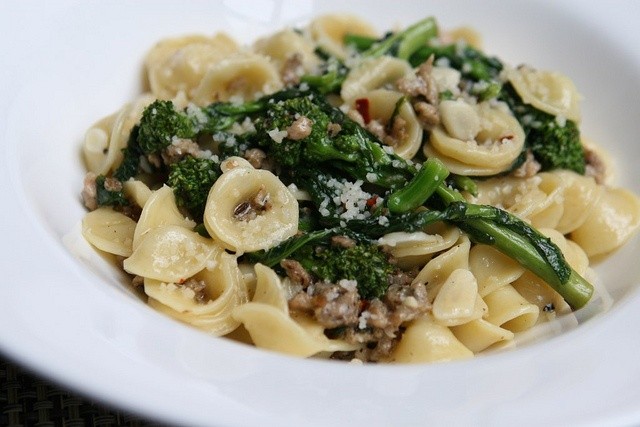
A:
<box><xmin>0</xmin><ymin>0</ymin><xmax>640</xmax><ymax>427</ymax></box>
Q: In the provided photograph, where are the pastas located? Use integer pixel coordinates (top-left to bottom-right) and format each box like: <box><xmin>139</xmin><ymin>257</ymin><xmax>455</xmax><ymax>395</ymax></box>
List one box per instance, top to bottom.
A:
<box><xmin>77</xmin><ymin>10</ymin><xmax>640</xmax><ymax>366</ymax></box>
